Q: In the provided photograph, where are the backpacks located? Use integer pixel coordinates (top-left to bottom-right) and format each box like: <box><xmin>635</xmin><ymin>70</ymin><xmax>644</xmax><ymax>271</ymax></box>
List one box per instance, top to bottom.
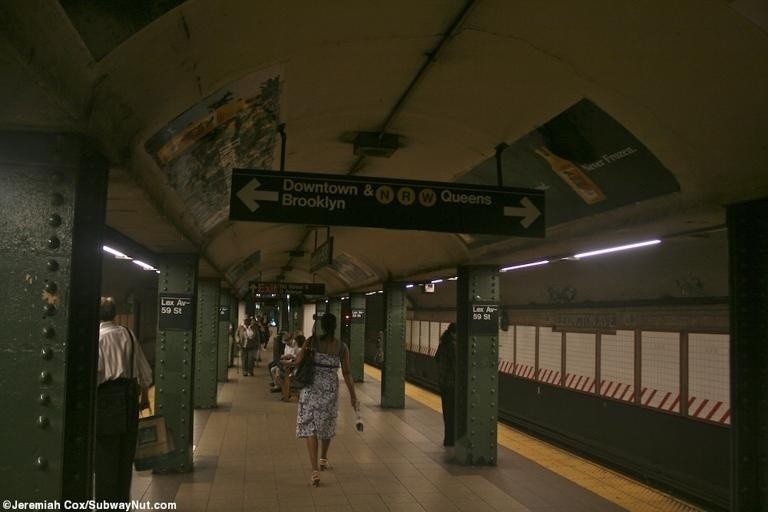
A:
<box><xmin>246</xmin><ymin>338</ymin><xmax>258</xmax><ymax>352</ymax></box>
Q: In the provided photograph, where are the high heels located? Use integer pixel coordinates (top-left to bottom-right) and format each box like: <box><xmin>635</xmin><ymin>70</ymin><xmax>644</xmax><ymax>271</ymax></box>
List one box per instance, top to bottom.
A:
<box><xmin>319</xmin><ymin>457</ymin><xmax>330</xmax><ymax>471</ymax></box>
<box><xmin>310</xmin><ymin>469</ymin><xmax>320</xmax><ymax>488</ymax></box>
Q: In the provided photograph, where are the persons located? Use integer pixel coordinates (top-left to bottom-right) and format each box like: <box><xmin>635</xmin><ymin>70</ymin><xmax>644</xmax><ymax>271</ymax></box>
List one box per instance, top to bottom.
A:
<box><xmin>95</xmin><ymin>294</ymin><xmax>157</xmax><ymax>511</ymax></box>
<box><xmin>228</xmin><ymin>309</ymin><xmax>305</xmax><ymax>401</ymax></box>
<box><xmin>292</xmin><ymin>312</ymin><xmax>360</xmax><ymax>486</ymax></box>
<box><xmin>435</xmin><ymin>321</ymin><xmax>457</xmax><ymax>447</ymax></box>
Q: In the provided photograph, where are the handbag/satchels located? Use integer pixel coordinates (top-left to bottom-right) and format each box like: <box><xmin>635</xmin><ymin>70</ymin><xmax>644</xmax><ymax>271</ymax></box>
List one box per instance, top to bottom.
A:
<box><xmin>288</xmin><ymin>335</ymin><xmax>317</xmax><ymax>393</ymax></box>
<box><xmin>96</xmin><ymin>375</ymin><xmax>139</xmax><ymax>441</ymax></box>
<box><xmin>130</xmin><ymin>405</ymin><xmax>175</xmax><ymax>473</ymax></box>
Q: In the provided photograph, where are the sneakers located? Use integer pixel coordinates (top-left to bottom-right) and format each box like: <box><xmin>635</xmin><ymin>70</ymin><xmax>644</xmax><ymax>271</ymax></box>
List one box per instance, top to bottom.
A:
<box><xmin>242</xmin><ymin>369</ymin><xmax>255</xmax><ymax>376</ymax></box>
<box><xmin>267</xmin><ymin>379</ymin><xmax>294</xmax><ymax>402</ymax></box>
